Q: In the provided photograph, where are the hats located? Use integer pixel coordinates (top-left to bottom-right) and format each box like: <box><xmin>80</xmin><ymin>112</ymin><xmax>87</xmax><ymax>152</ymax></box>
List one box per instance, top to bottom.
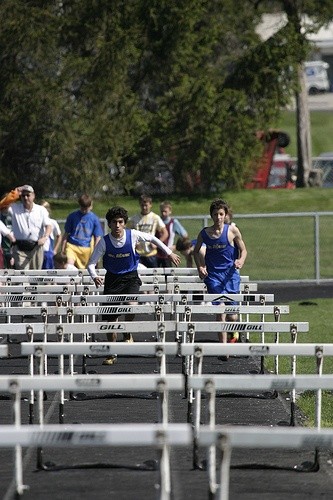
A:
<box><xmin>21</xmin><ymin>185</ymin><xmax>34</xmax><ymax>193</ymax></box>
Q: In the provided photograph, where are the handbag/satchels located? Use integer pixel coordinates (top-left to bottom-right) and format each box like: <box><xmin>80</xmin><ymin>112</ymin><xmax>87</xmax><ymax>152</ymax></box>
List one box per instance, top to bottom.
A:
<box><xmin>16</xmin><ymin>239</ymin><xmax>36</xmax><ymax>251</ymax></box>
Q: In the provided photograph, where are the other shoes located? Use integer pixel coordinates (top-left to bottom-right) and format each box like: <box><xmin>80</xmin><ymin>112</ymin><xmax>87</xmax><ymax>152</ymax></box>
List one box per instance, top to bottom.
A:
<box><xmin>228</xmin><ymin>332</ymin><xmax>239</xmax><ymax>343</ymax></box>
<box><xmin>121</xmin><ymin>334</ymin><xmax>133</xmax><ymax>343</ymax></box>
<box><xmin>102</xmin><ymin>354</ymin><xmax>117</xmax><ymax>365</ymax></box>
<box><xmin>218</xmin><ymin>355</ymin><xmax>229</xmax><ymax>361</ymax></box>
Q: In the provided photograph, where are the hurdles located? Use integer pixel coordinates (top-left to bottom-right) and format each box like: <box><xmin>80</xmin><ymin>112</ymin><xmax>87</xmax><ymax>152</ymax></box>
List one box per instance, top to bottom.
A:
<box><xmin>0</xmin><ymin>268</ymin><xmax>333</xmax><ymax>500</ymax></box>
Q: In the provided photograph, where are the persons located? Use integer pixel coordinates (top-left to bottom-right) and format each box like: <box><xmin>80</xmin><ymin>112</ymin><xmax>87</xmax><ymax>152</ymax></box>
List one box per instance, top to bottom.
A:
<box><xmin>0</xmin><ymin>185</ymin><xmax>238</xmax><ymax>306</ymax></box>
<box><xmin>195</xmin><ymin>199</ymin><xmax>248</xmax><ymax>361</ymax></box>
<box><xmin>86</xmin><ymin>206</ymin><xmax>181</xmax><ymax>365</ymax></box>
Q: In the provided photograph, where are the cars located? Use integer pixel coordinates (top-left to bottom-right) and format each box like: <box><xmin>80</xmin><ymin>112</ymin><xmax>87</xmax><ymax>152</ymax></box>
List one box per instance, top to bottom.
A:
<box><xmin>304</xmin><ymin>60</ymin><xmax>330</xmax><ymax>96</ymax></box>
<box><xmin>243</xmin><ymin>128</ymin><xmax>299</xmax><ymax>190</ymax></box>
<box><xmin>312</xmin><ymin>151</ymin><xmax>333</xmax><ymax>189</ymax></box>
